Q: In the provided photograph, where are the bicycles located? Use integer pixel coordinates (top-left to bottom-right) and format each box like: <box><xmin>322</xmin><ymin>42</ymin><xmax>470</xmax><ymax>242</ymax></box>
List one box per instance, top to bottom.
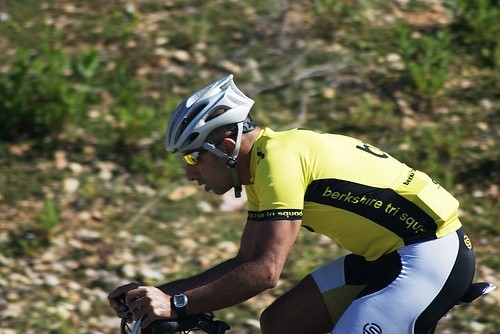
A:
<box><xmin>113</xmin><ymin>281</ymin><xmax>498</xmax><ymax>334</ymax></box>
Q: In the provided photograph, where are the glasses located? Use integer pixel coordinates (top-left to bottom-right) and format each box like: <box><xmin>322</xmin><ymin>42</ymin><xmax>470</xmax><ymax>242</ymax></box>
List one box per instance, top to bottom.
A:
<box><xmin>179</xmin><ymin>139</ymin><xmax>224</xmax><ymax>165</ymax></box>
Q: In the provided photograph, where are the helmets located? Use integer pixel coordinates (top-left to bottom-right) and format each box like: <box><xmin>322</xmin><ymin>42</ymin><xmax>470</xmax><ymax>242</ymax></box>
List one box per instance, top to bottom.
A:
<box><xmin>164</xmin><ymin>74</ymin><xmax>255</xmax><ymax>157</ymax></box>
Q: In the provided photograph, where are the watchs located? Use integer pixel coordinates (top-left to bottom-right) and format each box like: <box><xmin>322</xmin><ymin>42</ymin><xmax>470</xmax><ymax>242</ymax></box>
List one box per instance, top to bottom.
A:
<box><xmin>170</xmin><ymin>292</ymin><xmax>189</xmax><ymax>320</ymax></box>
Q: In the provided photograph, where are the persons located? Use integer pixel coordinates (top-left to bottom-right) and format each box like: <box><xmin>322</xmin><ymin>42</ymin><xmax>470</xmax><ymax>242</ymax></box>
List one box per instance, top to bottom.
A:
<box><xmin>106</xmin><ymin>74</ymin><xmax>475</xmax><ymax>334</ymax></box>
